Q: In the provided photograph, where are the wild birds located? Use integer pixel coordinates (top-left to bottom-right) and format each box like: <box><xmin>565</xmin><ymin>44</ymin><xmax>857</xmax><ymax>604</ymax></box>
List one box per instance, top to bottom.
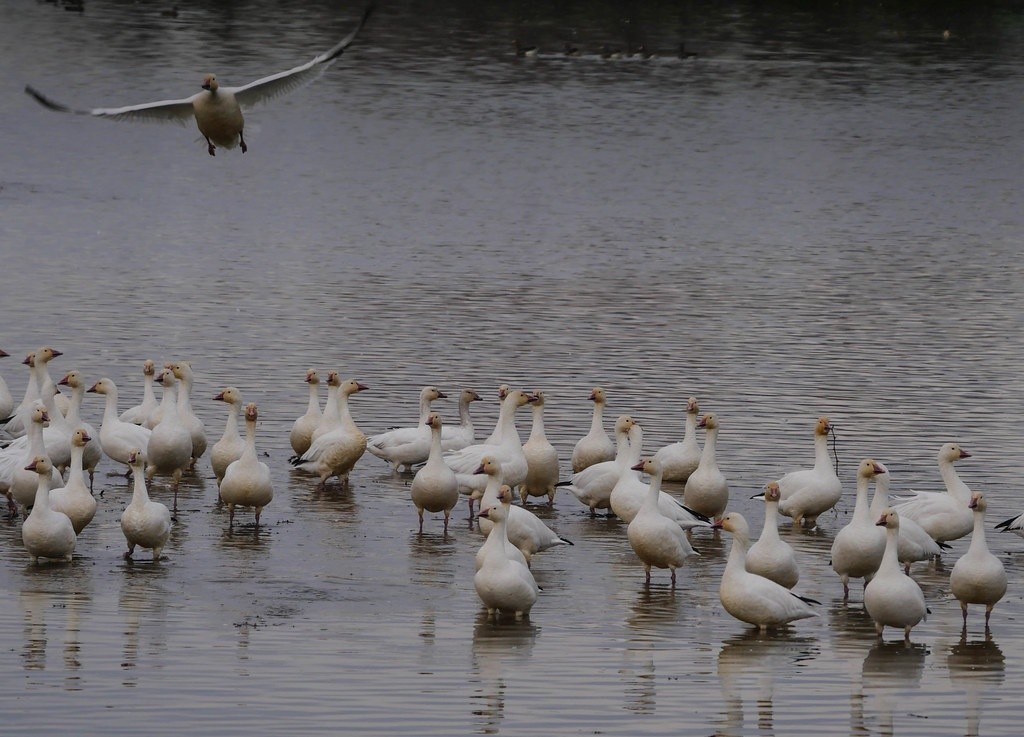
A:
<box><xmin>23</xmin><ymin>7</ymin><xmax>372</xmax><ymax>157</ymax></box>
<box><xmin>509</xmin><ymin>40</ymin><xmax>700</xmax><ymax>62</ymax></box>
<box><xmin>0</xmin><ymin>345</ymin><xmax>208</xmax><ymax>521</ymax></box>
<box><xmin>289</xmin><ymin>368</ymin><xmax>371</xmax><ymax>490</ymax></box>
<box><xmin>365</xmin><ymin>384</ymin><xmax>559</xmax><ymax>519</ymax></box>
<box><xmin>210</xmin><ymin>386</ymin><xmax>247</xmax><ymax>503</ymax></box>
<box><xmin>571</xmin><ymin>387</ymin><xmax>616</xmax><ymax>471</ymax></box>
<box><xmin>649</xmin><ymin>396</ymin><xmax>703</xmax><ymax>480</ymax></box>
<box><xmin>220</xmin><ymin>403</ymin><xmax>274</xmax><ymax>525</ymax></box>
<box><xmin>410</xmin><ymin>412</ymin><xmax>459</xmax><ymax>533</ymax></box>
<box><xmin>682</xmin><ymin>413</ymin><xmax>729</xmax><ymax>529</ymax></box>
<box><xmin>553</xmin><ymin>415</ymin><xmax>635</xmax><ymax>518</ymax></box>
<box><xmin>749</xmin><ymin>416</ymin><xmax>842</xmax><ymax>526</ymax></box>
<box><xmin>48</xmin><ymin>425</ymin><xmax>97</xmax><ymax>536</ymax></box>
<box><xmin>610</xmin><ymin>426</ymin><xmax>713</xmax><ymax>528</ymax></box>
<box><xmin>888</xmin><ymin>442</ymin><xmax>975</xmax><ymax>562</ymax></box>
<box><xmin>120</xmin><ymin>448</ymin><xmax>171</xmax><ymax>563</ymax></box>
<box><xmin>473</xmin><ymin>455</ymin><xmax>575</xmax><ymax>617</ymax></box>
<box><xmin>21</xmin><ymin>456</ymin><xmax>77</xmax><ymax>563</ymax></box>
<box><xmin>628</xmin><ymin>459</ymin><xmax>701</xmax><ymax>584</ymax></box>
<box><xmin>828</xmin><ymin>459</ymin><xmax>886</xmax><ymax>597</ymax></box>
<box><xmin>870</xmin><ymin>460</ymin><xmax>953</xmax><ymax>576</ymax></box>
<box><xmin>745</xmin><ymin>480</ymin><xmax>800</xmax><ymax>590</ymax></box>
<box><xmin>950</xmin><ymin>493</ymin><xmax>1005</xmax><ymax>631</ymax></box>
<box><xmin>862</xmin><ymin>513</ymin><xmax>932</xmax><ymax>648</ymax></box>
<box><xmin>710</xmin><ymin>515</ymin><xmax>823</xmax><ymax>635</ymax></box>
